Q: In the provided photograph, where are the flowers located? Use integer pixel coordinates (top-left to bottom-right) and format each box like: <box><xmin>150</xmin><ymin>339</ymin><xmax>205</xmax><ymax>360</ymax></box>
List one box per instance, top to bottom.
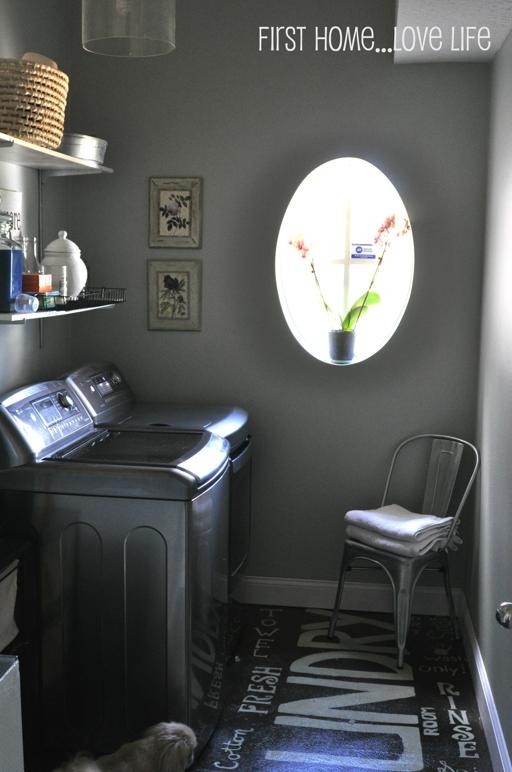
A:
<box><xmin>280</xmin><ymin>214</ymin><xmax>412</xmax><ymax>332</ymax></box>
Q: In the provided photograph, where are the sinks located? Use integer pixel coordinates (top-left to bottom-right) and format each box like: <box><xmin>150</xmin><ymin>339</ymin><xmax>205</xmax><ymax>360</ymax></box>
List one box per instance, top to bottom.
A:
<box><xmin>0</xmin><ymin>554</ymin><xmax>21</xmax><ymax>652</ymax></box>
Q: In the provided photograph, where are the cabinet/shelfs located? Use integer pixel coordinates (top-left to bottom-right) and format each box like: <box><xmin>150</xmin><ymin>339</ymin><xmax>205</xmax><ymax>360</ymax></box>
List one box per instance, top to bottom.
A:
<box><xmin>0</xmin><ymin>129</ymin><xmax>126</xmax><ymax>324</ymax></box>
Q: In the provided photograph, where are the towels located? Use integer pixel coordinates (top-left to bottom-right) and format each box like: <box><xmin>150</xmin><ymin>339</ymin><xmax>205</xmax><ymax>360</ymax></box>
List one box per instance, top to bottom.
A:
<box><xmin>345</xmin><ymin>504</ymin><xmax>465</xmax><ymax>561</ymax></box>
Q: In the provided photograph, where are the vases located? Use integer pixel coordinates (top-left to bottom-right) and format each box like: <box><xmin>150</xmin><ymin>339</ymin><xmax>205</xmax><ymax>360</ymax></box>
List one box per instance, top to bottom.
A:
<box><xmin>328</xmin><ymin>328</ymin><xmax>359</xmax><ymax>364</ymax></box>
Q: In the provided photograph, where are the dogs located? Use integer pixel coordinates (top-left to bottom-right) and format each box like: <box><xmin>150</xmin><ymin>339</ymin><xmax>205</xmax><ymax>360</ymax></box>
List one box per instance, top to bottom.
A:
<box><xmin>57</xmin><ymin>720</ymin><xmax>199</xmax><ymax>772</ymax></box>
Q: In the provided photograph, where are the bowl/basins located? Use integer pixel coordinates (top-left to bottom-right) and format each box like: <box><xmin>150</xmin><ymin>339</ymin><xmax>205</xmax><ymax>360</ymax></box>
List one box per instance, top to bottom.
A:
<box><xmin>51</xmin><ymin>130</ymin><xmax>109</xmax><ymax>164</ymax></box>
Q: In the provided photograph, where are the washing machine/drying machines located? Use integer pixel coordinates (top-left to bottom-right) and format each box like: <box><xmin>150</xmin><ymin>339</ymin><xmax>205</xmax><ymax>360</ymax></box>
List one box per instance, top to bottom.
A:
<box><xmin>66</xmin><ymin>359</ymin><xmax>255</xmax><ymax>663</ymax></box>
<box><xmin>0</xmin><ymin>378</ymin><xmax>229</xmax><ymax>758</ymax></box>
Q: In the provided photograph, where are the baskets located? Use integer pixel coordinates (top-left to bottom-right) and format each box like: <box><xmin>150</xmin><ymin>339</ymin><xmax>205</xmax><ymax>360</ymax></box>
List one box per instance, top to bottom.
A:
<box><xmin>0</xmin><ymin>50</ymin><xmax>71</xmax><ymax>149</ymax></box>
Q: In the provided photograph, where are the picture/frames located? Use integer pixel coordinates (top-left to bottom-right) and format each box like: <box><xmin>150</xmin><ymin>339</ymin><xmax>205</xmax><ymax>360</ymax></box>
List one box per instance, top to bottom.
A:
<box><xmin>146</xmin><ymin>175</ymin><xmax>205</xmax><ymax>333</ymax></box>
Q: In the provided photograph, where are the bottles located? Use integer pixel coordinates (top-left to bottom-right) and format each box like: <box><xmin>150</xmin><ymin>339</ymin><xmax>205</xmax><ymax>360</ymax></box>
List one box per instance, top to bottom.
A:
<box><xmin>0</xmin><ymin>214</ymin><xmax>88</xmax><ymax>314</ymax></box>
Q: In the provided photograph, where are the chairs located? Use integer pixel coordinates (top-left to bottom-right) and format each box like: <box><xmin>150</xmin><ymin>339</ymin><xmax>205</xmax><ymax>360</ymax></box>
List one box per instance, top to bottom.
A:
<box><xmin>328</xmin><ymin>433</ymin><xmax>480</xmax><ymax>669</ymax></box>
